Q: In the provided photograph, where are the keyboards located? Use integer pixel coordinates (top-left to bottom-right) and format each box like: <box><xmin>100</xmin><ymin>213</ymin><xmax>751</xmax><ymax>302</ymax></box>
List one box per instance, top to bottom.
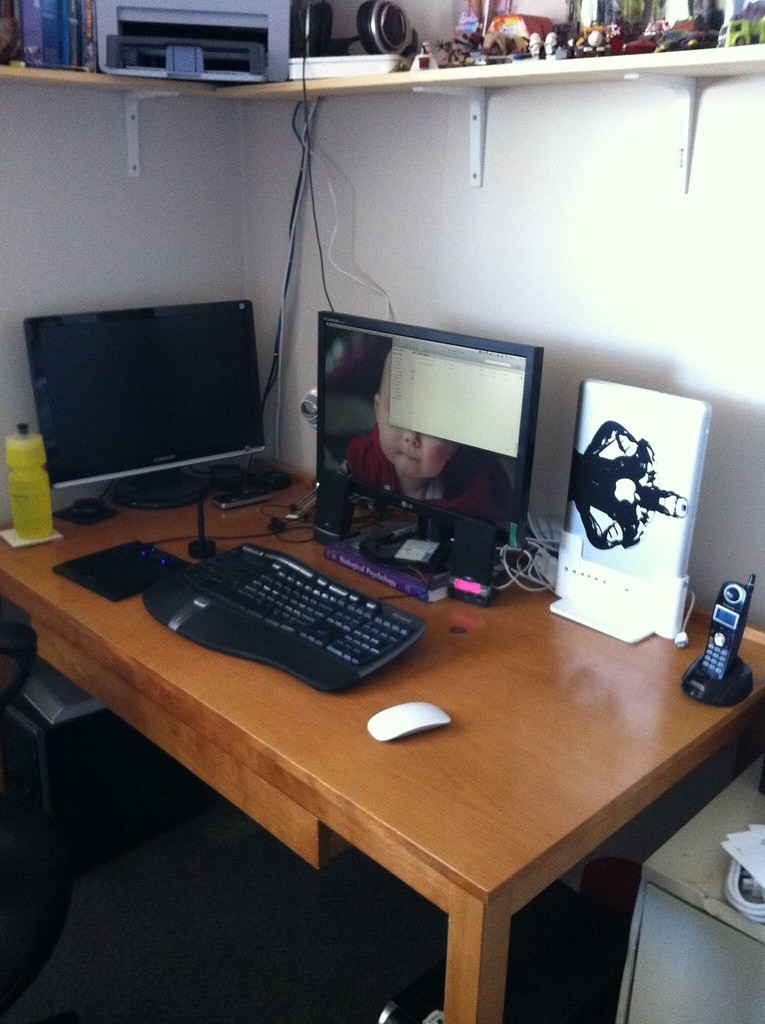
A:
<box><xmin>143</xmin><ymin>544</ymin><xmax>427</xmax><ymax>692</ymax></box>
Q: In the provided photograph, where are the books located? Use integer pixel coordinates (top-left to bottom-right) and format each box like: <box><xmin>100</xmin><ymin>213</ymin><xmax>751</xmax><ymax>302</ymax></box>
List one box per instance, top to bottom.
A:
<box><xmin>324</xmin><ymin>522</ymin><xmax>447</xmax><ymax>603</ymax></box>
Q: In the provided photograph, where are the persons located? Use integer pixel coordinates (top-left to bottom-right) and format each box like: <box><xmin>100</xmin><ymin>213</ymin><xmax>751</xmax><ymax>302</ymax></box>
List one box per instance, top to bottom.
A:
<box><xmin>345</xmin><ymin>349</ymin><xmax>468</xmax><ymax>500</ymax></box>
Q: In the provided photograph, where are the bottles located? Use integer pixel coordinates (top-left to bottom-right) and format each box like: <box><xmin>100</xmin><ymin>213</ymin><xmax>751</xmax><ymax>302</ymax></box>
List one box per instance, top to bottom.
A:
<box><xmin>4</xmin><ymin>423</ymin><xmax>53</xmax><ymax>540</ymax></box>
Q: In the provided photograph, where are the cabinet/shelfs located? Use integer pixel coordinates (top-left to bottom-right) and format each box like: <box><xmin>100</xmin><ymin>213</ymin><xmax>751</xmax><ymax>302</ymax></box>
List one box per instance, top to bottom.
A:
<box><xmin>615</xmin><ymin>751</ymin><xmax>765</xmax><ymax>1024</ymax></box>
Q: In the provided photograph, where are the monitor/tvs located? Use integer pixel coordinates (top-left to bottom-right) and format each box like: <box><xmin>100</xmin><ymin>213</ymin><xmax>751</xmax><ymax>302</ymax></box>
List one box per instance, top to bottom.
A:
<box><xmin>24</xmin><ymin>301</ymin><xmax>266</xmax><ymax>510</ymax></box>
<box><xmin>316</xmin><ymin>310</ymin><xmax>545</xmax><ymax>586</ymax></box>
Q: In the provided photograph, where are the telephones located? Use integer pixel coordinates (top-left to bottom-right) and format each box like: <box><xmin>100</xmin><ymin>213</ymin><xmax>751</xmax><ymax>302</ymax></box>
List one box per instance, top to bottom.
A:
<box><xmin>679</xmin><ymin>572</ymin><xmax>756</xmax><ymax>707</ymax></box>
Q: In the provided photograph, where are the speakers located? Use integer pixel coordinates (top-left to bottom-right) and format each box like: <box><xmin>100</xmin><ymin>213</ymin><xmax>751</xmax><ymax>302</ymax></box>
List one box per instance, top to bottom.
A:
<box><xmin>313</xmin><ymin>471</ymin><xmax>358</xmax><ymax>545</ymax></box>
<box><xmin>449</xmin><ymin>525</ymin><xmax>499</xmax><ymax>607</ymax></box>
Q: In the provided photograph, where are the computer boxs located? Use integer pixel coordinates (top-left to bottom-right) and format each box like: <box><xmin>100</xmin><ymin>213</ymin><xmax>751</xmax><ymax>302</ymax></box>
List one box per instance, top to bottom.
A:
<box><xmin>0</xmin><ymin>656</ymin><xmax>224</xmax><ymax>880</ymax></box>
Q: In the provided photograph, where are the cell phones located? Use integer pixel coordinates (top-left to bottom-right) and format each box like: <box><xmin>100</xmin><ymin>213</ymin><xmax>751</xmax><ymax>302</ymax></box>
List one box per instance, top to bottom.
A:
<box><xmin>212</xmin><ymin>486</ymin><xmax>272</xmax><ymax>509</ymax></box>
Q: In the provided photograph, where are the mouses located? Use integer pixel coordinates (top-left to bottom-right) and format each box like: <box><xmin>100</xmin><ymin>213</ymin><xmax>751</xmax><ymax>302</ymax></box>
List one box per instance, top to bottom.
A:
<box><xmin>367</xmin><ymin>701</ymin><xmax>451</xmax><ymax>742</ymax></box>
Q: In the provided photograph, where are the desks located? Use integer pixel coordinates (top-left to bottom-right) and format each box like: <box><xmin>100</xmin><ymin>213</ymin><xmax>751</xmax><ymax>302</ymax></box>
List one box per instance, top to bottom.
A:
<box><xmin>1</xmin><ymin>459</ymin><xmax>765</xmax><ymax>1024</ymax></box>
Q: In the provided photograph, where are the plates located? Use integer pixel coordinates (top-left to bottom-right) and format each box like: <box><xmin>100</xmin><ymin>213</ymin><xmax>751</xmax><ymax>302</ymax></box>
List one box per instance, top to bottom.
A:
<box><xmin>289</xmin><ymin>54</ymin><xmax>410</xmax><ymax>82</ymax></box>
